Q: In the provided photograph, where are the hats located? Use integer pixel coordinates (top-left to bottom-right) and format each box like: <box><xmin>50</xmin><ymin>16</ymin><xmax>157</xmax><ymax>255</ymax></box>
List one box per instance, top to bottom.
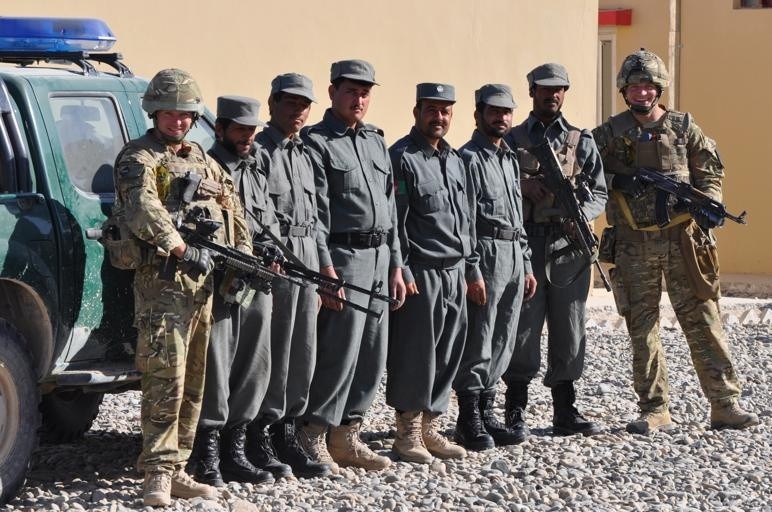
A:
<box><xmin>330</xmin><ymin>60</ymin><xmax>379</xmax><ymax>85</ymax></box>
<box><xmin>527</xmin><ymin>63</ymin><xmax>569</xmax><ymax>90</ymax></box>
<box><xmin>270</xmin><ymin>73</ymin><xmax>318</xmax><ymax>104</ymax></box>
<box><xmin>416</xmin><ymin>83</ymin><xmax>457</xmax><ymax>103</ymax></box>
<box><xmin>474</xmin><ymin>84</ymin><xmax>516</xmax><ymax>109</ymax></box>
<box><xmin>219</xmin><ymin>96</ymin><xmax>262</xmax><ymax>127</ymax></box>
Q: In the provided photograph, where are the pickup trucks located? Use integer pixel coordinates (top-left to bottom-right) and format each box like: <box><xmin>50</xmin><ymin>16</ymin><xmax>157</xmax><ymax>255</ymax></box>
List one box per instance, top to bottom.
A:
<box><xmin>0</xmin><ymin>14</ymin><xmax>216</xmax><ymax>499</ymax></box>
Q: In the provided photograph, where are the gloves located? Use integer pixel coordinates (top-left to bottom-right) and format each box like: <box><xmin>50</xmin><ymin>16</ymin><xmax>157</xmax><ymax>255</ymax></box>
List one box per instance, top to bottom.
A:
<box><xmin>612</xmin><ymin>174</ymin><xmax>647</xmax><ymax>198</ymax></box>
<box><xmin>696</xmin><ymin>209</ymin><xmax>714</xmax><ymax>229</ymax></box>
<box><xmin>178</xmin><ymin>246</ymin><xmax>213</xmax><ymax>276</ymax></box>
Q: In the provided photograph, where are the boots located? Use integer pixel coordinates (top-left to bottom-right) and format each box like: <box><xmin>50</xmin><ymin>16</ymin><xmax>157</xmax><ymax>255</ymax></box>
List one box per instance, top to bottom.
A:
<box><xmin>552</xmin><ymin>383</ymin><xmax>600</xmax><ymax>435</ymax></box>
<box><xmin>504</xmin><ymin>387</ymin><xmax>528</xmax><ymax>435</ymax></box>
<box><xmin>479</xmin><ymin>388</ymin><xmax>525</xmax><ymax>446</ymax></box>
<box><xmin>453</xmin><ymin>391</ymin><xmax>494</xmax><ymax>451</ymax></box>
<box><xmin>393</xmin><ymin>410</ymin><xmax>432</xmax><ymax>465</ymax></box>
<box><xmin>422</xmin><ymin>412</ymin><xmax>468</xmax><ymax>459</ymax></box>
<box><xmin>193</xmin><ymin>420</ymin><xmax>392</xmax><ymax>487</ymax></box>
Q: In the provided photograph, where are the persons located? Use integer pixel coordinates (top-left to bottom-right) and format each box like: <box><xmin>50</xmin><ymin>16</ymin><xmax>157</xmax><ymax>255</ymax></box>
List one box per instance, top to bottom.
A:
<box><xmin>591</xmin><ymin>48</ymin><xmax>761</xmax><ymax>433</ymax></box>
<box><xmin>300</xmin><ymin>59</ymin><xmax>408</xmax><ymax>474</ymax></box>
<box><xmin>504</xmin><ymin>63</ymin><xmax>609</xmax><ymax>438</ymax></box>
<box><xmin>110</xmin><ymin>69</ymin><xmax>254</xmax><ymax>508</ymax></box>
<box><xmin>246</xmin><ymin>72</ymin><xmax>330</xmax><ymax>482</ymax></box>
<box><xmin>388</xmin><ymin>81</ymin><xmax>473</xmax><ymax>464</ymax></box>
<box><xmin>458</xmin><ymin>83</ymin><xmax>539</xmax><ymax>451</ymax></box>
<box><xmin>191</xmin><ymin>96</ymin><xmax>293</xmax><ymax>487</ymax></box>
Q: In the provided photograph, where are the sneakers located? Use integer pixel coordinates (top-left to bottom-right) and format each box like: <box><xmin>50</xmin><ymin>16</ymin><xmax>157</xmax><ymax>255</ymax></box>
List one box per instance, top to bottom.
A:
<box><xmin>710</xmin><ymin>397</ymin><xmax>759</xmax><ymax>429</ymax></box>
<box><xmin>626</xmin><ymin>410</ymin><xmax>671</xmax><ymax>434</ymax></box>
<box><xmin>172</xmin><ymin>470</ymin><xmax>216</xmax><ymax>499</ymax></box>
<box><xmin>144</xmin><ymin>472</ymin><xmax>171</xmax><ymax>506</ymax></box>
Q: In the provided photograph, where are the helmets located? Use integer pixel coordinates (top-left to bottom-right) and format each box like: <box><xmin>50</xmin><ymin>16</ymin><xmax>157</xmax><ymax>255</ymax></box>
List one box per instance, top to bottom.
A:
<box><xmin>617</xmin><ymin>50</ymin><xmax>669</xmax><ymax>88</ymax></box>
<box><xmin>142</xmin><ymin>69</ymin><xmax>205</xmax><ymax>115</ymax></box>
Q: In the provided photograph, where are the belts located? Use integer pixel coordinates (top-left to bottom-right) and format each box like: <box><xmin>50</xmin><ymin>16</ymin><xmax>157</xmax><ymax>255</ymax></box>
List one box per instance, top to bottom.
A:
<box><xmin>524</xmin><ymin>223</ymin><xmax>564</xmax><ymax>237</ymax></box>
<box><xmin>329</xmin><ymin>233</ymin><xmax>388</xmax><ymax>249</ymax></box>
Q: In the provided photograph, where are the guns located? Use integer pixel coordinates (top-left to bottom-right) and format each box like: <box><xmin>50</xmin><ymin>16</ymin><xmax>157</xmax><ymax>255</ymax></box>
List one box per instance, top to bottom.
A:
<box><xmin>530</xmin><ymin>138</ymin><xmax>612</xmax><ymax>293</ymax></box>
<box><xmin>603</xmin><ymin>154</ymin><xmax>747</xmax><ymax>228</ymax></box>
<box><xmin>155</xmin><ymin>221</ymin><xmax>384</xmax><ymax>323</ymax></box>
<box><xmin>251</xmin><ymin>241</ymin><xmax>401</xmax><ymax>307</ymax></box>
